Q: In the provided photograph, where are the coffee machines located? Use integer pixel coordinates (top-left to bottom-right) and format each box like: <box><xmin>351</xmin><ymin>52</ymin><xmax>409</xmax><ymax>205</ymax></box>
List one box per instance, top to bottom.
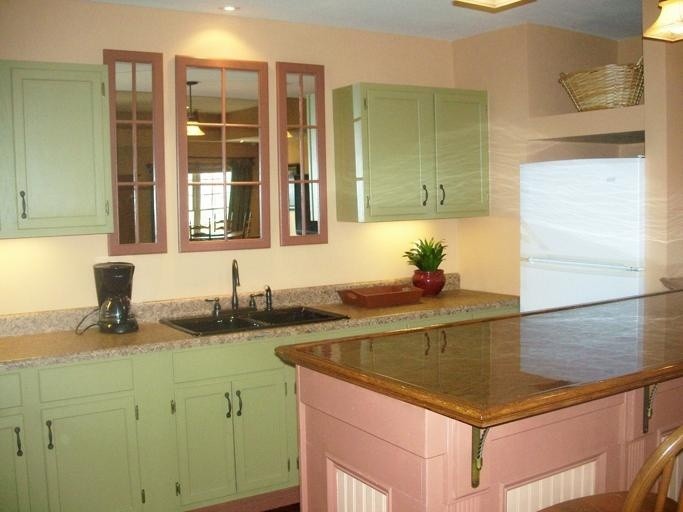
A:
<box><xmin>92</xmin><ymin>262</ymin><xmax>139</xmax><ymax>333</ymax></box>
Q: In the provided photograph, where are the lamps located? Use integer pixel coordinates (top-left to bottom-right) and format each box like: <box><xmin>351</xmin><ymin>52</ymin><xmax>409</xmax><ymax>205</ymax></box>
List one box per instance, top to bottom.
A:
<box><xmin>642</xmin><ymin>0</ymin><xmax>682</xmax><ymax>43</ymax></box>
<box><xmin>452</xmin><ymin>1</ymin><xmax>534</xmax><ymax>15</ymax></box>
<box><xmin>183</xmin><ymin>112</ymin><xmax>207</xmax><ymax>138</ymax></box>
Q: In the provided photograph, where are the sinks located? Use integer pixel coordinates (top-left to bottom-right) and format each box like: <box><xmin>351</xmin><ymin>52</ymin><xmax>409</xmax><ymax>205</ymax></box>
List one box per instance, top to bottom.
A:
<box><xmin>235</xmin><ymin>305</ymin><xmax>350</xmax><ymax>329</ymax></box>
<box><xmin>159</xmin><ymin>313</ymin><xmax>259</xmax><ymax>336</ymax></box>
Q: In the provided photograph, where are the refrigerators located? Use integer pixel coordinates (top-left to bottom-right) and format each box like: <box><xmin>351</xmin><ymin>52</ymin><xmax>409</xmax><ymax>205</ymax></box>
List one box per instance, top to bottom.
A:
<box><xmin>520</xmin><ymin>155</ymin><xmax>644</xmax><ymax>311</ymax></box>
<box><xmin>519</xmin><ymin>298</ymin><xmax>645</xmax><ymax>382</ymax></box>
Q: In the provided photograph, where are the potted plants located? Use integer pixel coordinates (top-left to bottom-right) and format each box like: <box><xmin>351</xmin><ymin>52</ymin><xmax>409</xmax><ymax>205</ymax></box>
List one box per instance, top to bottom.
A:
<box><xmin>403</xmin><ymin>235</ymin><xmax>449</xmax><ymax>296</ymax></box>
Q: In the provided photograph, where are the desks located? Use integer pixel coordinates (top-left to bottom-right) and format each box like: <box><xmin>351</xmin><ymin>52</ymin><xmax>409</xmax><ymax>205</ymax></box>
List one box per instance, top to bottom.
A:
<box><xmin>276</xmin><ymin>289</ymin><xmax>683</xmax><ymax>512</ymax></box>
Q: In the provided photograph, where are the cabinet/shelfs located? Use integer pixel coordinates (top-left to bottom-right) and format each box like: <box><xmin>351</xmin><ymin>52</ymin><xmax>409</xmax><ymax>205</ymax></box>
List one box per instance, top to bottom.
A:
<box><xmin>0</xmin><ymin>58</ymin><xmax>114</xmax><ymax>239</ymax></box>
<box><xmin>332</xmin><ymin>82</ymin><xmax>490</xmax><ymax>224</ymax></box>
<box><xmin>166</xmin><ymin>339</ymin><xmax>294</xmax><ymax>512</ymax></box>
<box><xmin>0</xmin><ymin>358</ymin><xmax>147</xmax><ymax>512</ymax></box>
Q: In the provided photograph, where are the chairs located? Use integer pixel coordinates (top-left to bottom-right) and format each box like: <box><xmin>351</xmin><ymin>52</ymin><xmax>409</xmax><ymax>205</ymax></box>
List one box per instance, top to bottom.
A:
<box><xmin>189</xmin><ymin>218</ymin><xmax>214</xmax><ymax>238</ymax></box>
<box><xmin>214</xmin><ymin>218</ymin><xmax>233</xmax><ymax>241</ymax></box>
<box><xmin>537</xmin><ymin>425</ymin><xmax>683</xmax><ymax>512</ymax></box>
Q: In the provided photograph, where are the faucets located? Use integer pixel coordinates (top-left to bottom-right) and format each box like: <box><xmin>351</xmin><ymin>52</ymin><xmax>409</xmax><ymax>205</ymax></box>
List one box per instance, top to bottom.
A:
<box><xmin>231</xmin><ymin>259</ymin><xmax>241</xmax><ymax>313</ymax></box>
<box><xmin>265</xmin><ymin>286</ymin><xmax>272</xmax><ymax>311</ymax></box>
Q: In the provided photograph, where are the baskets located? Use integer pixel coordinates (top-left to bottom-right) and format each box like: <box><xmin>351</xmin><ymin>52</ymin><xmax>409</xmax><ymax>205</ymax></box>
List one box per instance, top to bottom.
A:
<box><xmin>558</xmin><ymin>55</ymin><xmax>644</xmax><ymax>112</ymax></box>
<box><xmin>336</xmin><ymin>284</ymin><xmax>425</xmax><ymax>309</ymax></box>
